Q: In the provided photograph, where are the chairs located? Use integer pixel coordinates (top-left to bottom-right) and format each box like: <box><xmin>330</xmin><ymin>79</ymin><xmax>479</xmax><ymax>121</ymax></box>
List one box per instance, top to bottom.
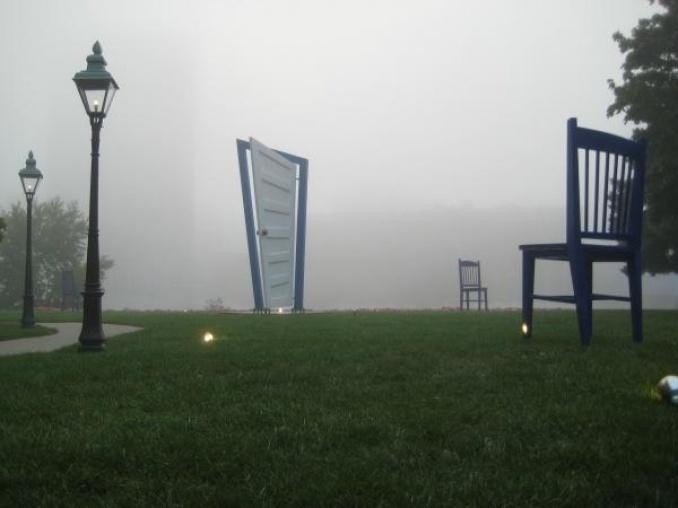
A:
<box><xmin>519</xmin><ymin>117</ymin><xmax>647</xmax><ymax>345</ymax></box>
<box><xmin>456</xmin><ymin>257</ymin><xmax>490</xmax><ymax>312</ymax></box>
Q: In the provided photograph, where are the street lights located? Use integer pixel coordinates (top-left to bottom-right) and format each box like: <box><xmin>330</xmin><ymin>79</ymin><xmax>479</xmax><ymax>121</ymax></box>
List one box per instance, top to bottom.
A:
<box><xmin>71</xmin><ymin>40</ymin><xmax>119</xmax><ymax>351</ymax></box>
<box><xmin>18</xmin><ymin>152</ymin><xmax>44</xmax><ymax>326</ymax></box>
